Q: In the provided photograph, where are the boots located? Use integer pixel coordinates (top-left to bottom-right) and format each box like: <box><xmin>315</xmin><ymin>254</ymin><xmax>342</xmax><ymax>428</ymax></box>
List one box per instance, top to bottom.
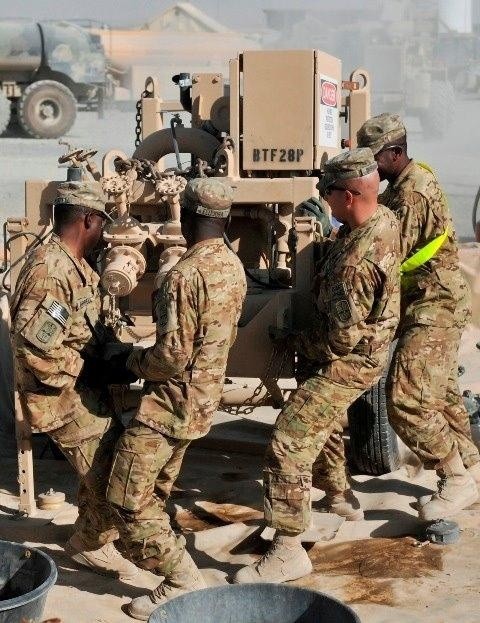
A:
<box><xmin>415</xmin><ymin>461</ymin><xmax>480</xmax><ymax>527</ymax></box>
<box><xmin>305</xmin><ymin>490</ymin><xmax>366</xmax><ymax>522</ymax></box>
<box><xmin>63</xmin><ymin>523</ymin><xmax>139</xmax><ymax>580</ymax></box>
<box><xmin>232</xmin><ymin>530</ymin><xmax>317</xmax><ymax>588</ymax></box>
<box><xmin>126</xmin><ymin>550</ymin><xmax>209</xmax><ymax>623</ymax></box>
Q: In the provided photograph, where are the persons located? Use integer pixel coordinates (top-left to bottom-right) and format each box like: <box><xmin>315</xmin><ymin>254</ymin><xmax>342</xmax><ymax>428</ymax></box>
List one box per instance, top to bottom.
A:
<box><xmin>299</xmin><ymin>112</ymin><xmax>480</xmax><ymax>520</ymax></box>
<box><xmin>233</xmin><ymin>147</ymin><xmax>403</xmax><ymax>583</ymax></box>
<box><xmin>107</xmin><ymin>176</ymin><xmax>247</xmax><ymax>622</ymax></box>
<box><xmin>10</xmin><ymin>180</ymin><xmax>139</xmax><ymax>578</ymax></box>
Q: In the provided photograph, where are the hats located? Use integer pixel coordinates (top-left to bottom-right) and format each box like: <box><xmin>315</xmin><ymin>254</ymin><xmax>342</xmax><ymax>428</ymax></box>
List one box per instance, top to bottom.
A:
<box><xmin>356</xmin><ymin>111</ymin><xmax>408</xmax><ymax>156</ymax></box>
<box><xmin>318</xmin><ymin>146</ymin><xmax>379</xmax><ymax>181</ymax></box>
<box><xmin>177</xmin><ymin>177</ymin><xmax>236</xmax><ymax>219</ymax></box>
<box><xmin>51</xmin><ymin>179</ymin><xmax>118</xmax><ymax>225</ymax></box>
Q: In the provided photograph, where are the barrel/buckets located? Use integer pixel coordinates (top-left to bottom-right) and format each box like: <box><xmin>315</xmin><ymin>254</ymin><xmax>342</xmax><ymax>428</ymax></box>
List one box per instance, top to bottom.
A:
<box><xmin>0</xmin><ymin>539</ymin><xmax>58</xmax><ymax>623</ymax></box>
<box><xmin>147</xmin><ymin>582</ymin><xmax>362</xmax><ymax>623</ymax></box>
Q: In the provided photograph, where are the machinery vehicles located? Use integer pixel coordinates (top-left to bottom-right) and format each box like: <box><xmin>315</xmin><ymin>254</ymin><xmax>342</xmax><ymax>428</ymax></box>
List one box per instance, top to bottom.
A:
<box><xmin>260</xmin><ymin>1</ymin><xmax>458</xmax><ymax>140</ymax></box>
<box><xmin>0</xmin><ymin>14</ymin><xmax>120</xmax><ymax>139</ymax></box>
